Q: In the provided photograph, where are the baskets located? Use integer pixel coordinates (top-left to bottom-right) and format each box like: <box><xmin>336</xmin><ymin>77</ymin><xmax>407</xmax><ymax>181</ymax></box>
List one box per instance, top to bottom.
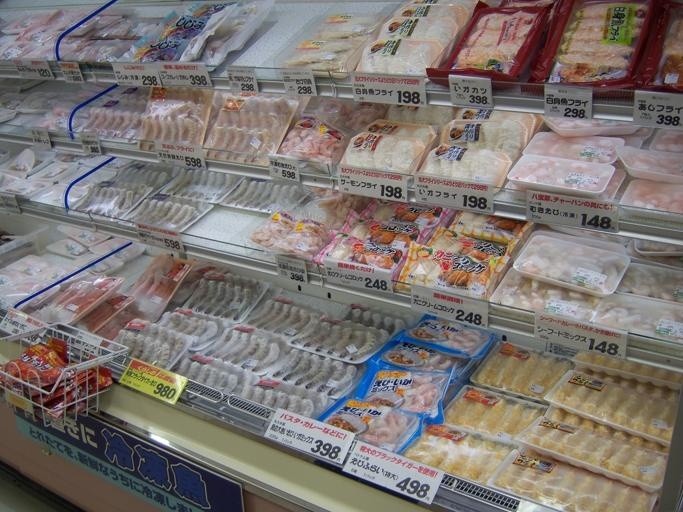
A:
<box><xmin>1</xmin><ymin>321</ymin><xmax>132</xmax><ymax>426</ymax></box>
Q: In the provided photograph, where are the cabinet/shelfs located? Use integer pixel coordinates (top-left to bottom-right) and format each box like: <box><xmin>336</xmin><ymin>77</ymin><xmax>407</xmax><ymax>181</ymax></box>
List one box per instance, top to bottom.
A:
<box><xmin>0</xmin><ymin>2</ymin><xmax>683</xmax><ymax>512</ymax></box>
